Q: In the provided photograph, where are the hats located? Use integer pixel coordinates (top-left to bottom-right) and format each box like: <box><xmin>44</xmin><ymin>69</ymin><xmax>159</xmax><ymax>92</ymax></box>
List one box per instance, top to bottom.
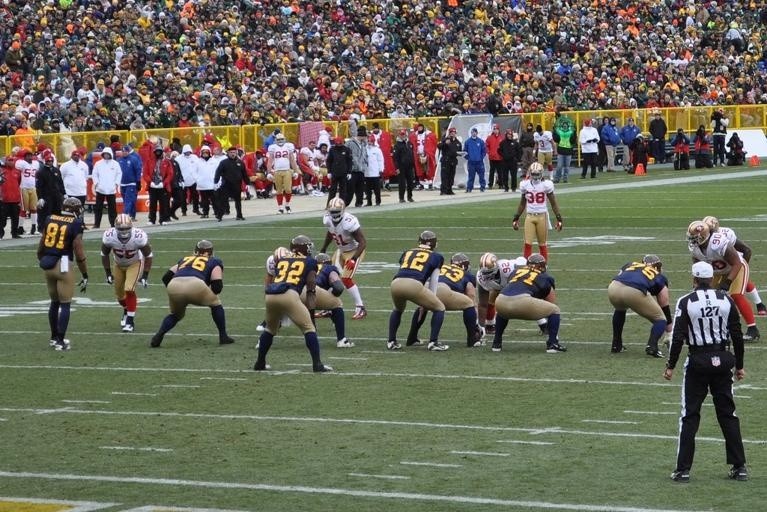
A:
<box><xmin>0</xmin><ymin>0</ymin><xmax>767</xmax><ymax>127</ymax></box>
<box><xmin>637</xmin><ymin>125</ymin><xmax>738</xmax><ymax>142</ymax></box>
<box><xmin>448</xmin><ymin>126</ymin><xmax>544</xmax><ymax>137</ymax></box>
<box><xmin>322</xmin><ymin>127</ymin><xmax>424</xmax><ymax>145</ymax></box>
<box><xmin>256</xmin><ymin>129</ymin><xmax>328</xmax><ymax>163</ymax></box>
<box><xmin>147</xmin><ymin>132</ymin><xmax>246</xmax><ymax>158</ymax></box>
<box><xmin>71</xmin><ymin>134</ymin><xmax>134</xmax><ymax>160</ymax></box>
<box><xmin>0</xmin><ymin>143</ymin><xmax>55</xmax><ymax>166</ymax></box>
<box><xmin>691</xmin><ymin>261</ymin><xmax>715</xmax><ymax>279</ymax></box>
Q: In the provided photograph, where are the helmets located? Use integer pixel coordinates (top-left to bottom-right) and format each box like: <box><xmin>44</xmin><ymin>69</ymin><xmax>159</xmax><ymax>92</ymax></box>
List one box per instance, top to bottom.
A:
<box><xmin>326</xmin><ymin>196</ymin><xmax>347</xmax><ymax>226</ymax></box>
<box><xmin>703</xmin><ymin>215</ymin><xmax>719</xmax><ymax>230</ymax></box>
<box><xmin>686</xmin><ymin>220</ymin><xmax>711</xmax><ymax>246</ymax></box>
<box><xmin>418</xmin><ymin>228</ymin><xmax>439</xmax><ymax>250</ymax></box>
<box><xmin>287</xmin><ymin>232</ymin><xmax>334</xmax><ymax>268</ymax></box>
<box><xmin>194</xmin><ymin>239</ymin><xmax>214</xmax><ymax>257</ymax></box>
<box><xmin>478</xmin><ymin>249</ymin><xmax>550</xmax><ymax>278</ymax></box>
<box><xmin>449</xmin><ymin>250</ymin><xmax>472</xmax><ymax>271</ymax></box>
<box><xmin>642</xmin><ymin>253</ymin><xmax>661</xmax><ymax>268</ymax></box>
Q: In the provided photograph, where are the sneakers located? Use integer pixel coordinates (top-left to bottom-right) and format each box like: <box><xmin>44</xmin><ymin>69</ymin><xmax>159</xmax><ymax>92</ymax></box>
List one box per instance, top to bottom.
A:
<box><xmin>276</xmin><ymin>206</ymin><xmax>293</xmax><ymax>215</ymax></box>
<box><xmin>0</xmin><ymin>208</ymin><xmax>246</xmax><ymax>242</ymax></box>
<box><xmin>610</xmin><ymin>304</ymin><xmax>767</xmax><ymax>359</ymax></box>
<box><xmin>254</xmin><ymin>305</ymin><xmax>368</xmax><ymax>372</ymax></box>
<box><xmin>120</xmin><ymin>315</ymin><xmax>135</xmax><ymax>332</ymax></box>
<box><xmin>387</xmin><ymin>324</ymin><xmax>567</xmax><ymax>354</ymax></box>
<box><xmin>219</xmin><ymin>336</ymin><xmax>234</xmax><ymax>344</ymax></box>
<box><xmin>150</xmin><ymin>337</ymin><xmax>163</xmax><ymax>348</ymax></box>
<box><xmin>49</xmin><ymin>338</ymin><xmax>71</xmax><ymax>351</ymax></box>
<box><xmin>727</xmin><ymin>464</ymin><xmax>749</xmax><ymax>481</ymax></box>
<box><xmin>670</xmin><ymin>469</ymin><xmax>690</xmax><ymax>483</ymax></box>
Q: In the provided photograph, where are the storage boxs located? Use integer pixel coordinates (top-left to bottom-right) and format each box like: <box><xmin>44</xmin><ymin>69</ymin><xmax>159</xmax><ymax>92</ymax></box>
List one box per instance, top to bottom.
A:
<box><xmin>84</xmin><ymin>150</ymin><xmax>160</xmax><ymax>214</ymax></box>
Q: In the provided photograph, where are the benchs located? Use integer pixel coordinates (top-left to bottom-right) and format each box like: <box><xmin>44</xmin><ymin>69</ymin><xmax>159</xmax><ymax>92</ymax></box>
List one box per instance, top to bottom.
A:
<box><xmin>663</xmin><ymin>137</ymin><xmax>720</xmax><ymax>158</ymax></box>
<box><xmin>551</xmin><ymin>144</ymin><xmax>586</xmax><ymax>166</ymax></box>
<box><xmin>613</xmin><ymin>144</ymin><xmax>630</xmax><ymax>156</ymax></box>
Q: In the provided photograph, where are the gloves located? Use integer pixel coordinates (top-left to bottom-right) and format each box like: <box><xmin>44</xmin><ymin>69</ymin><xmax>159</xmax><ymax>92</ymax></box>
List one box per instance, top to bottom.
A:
<box><xmin>510</xmin><ymin>212</ymin><xmax>521</xmax><ymax>231</ymax></box>
<box><xmin>553</xmin><ymin>213</ymin><xmax>564</xmax><ymax>231</ymax></box>
<box><xmin>345</xmin><ymin>255</ymin><xmax>357</xmax><ymax>270</ymax></box>
<box><xmin>104</xmin><ymin>268</ymin><xmax>117</xmax><ymax>284</ymax></box>
<box><xmin>137</xmin><ymin>271</ymin><xmax>152</xmax><ymax>289</ymax></box>
<box><xmin>77</xmin><ymin>272</ymin><xmax>92</xmax><ymax>293</ymax></box>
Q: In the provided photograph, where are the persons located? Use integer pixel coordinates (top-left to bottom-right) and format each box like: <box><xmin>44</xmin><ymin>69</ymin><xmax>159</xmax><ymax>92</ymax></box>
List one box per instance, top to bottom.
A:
<box><xmin>511</xmin><ymin>163</ymin><xmax>563</xmax><ymax>269</ymax></box>
<box><xmin>312</xmin><ymin>195</ymin><xmax>369</xmax><ymax>321</ymax></box>
<box><xmin>36</xmin><ymin>197</ymin><xmax>88</xmax><ymax>352</ymax></box>
<box><xmin>99</xmin><ymin>212</ymin><xmax>153</xmax><ymax>332</ymax></box>
<box><xmin>701</xmin><ymin>214</ymin><xmax>766</xmax><ymax>318</ymax></box>
<box><xmin>686</xmin><ymin>220</ymin><xmax>760</xmax><ymax>343</ymax></box>
<box><xmin>385</xmin><ymin>230</ymin><xmax>452</xmax><ymax>351</ymax></box>
<box><xmin>252</xmin><ymin>235</ymin><xmax>335</xmax><ymax>372</ymax></box>
<box><xmin>150</xmin><ymin>240</ymin><xmax>234</xmax><ymax>350</ymax></box>
<box><xmin>255</xmin><ymin>246</ymin><xmax>294</xmax><ymax>332</ymax></box>
<box><xmin>254</xmin><ymin>252</ymin><xmax>355</xmax><ymax>348</ymax></box>
<box><xmin>406</xmin><ymin>252</ymin><xmax>485</xmax><ymax>347</ymax></box>
<box><xmin>477</xmin><ymin>252</ymin><xmax>556</xmax><ymax>336</ymax></box>
<box><xmin>490</xmin><ymin>253</ymin><xmax>571</xmax><ymax>354</ymax></box>
<box><xmin>606</xmin><ymin>253</ymin><xmax>673</xmax><ymax>359</ymax></box>
<box><xmin>661</xmin><ymin>261</ymin><xmax>749</xmax><ymax>483</ymax></box>
